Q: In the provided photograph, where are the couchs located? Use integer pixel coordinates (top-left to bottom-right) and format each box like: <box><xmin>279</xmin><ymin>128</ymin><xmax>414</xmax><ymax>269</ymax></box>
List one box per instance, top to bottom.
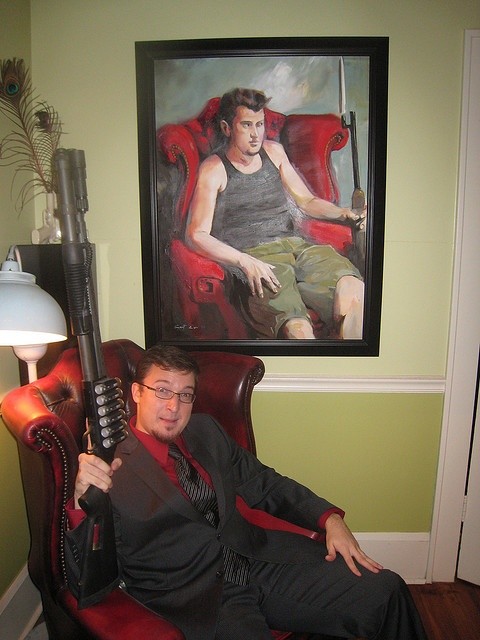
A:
<box><xmin>158</xmin><ymin>95</ymin><xmax>352</xmax><ymax>341</ymax></box>
<box><xmin>0</xmin><ymin>335</ymin><xmax>336</xmax><ymax>639</ymax></box>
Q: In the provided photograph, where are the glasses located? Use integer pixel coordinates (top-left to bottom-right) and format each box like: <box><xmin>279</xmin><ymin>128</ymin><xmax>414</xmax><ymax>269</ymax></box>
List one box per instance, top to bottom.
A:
<box><xmin>133</xmin><ymin>380</ymin><xmax>197</xmax><ymax>404</ymax></box>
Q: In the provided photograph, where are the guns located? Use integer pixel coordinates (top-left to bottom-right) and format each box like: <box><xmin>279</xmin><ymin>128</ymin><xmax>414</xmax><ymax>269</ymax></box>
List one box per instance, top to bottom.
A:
<box><xmin>337</xmin><ymin>55</ymin><xmax>367</xmax><ymax>272</ymax></box>
<box><xmin>51</xmin><ymin>146</ymin><xmax>129</xmax><ymax>609</ymax></box>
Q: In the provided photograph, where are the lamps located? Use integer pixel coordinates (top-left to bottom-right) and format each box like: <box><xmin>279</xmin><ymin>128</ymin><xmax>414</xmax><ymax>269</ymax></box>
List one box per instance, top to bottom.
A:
<box><xmin>0</xmin><ymin>246</ymin><xmax>69</xmax><ymax>382</ymax></box>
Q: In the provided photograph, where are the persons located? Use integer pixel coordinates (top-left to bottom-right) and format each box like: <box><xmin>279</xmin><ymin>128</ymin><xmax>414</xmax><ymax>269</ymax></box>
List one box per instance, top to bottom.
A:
<box><xmin>185</xmin><ymin>88</ymin><xmax>367</xmax><ymax>339</ymax></box>
<box><xmin>61</xmin><ymin>347</ymin><xmax>432</xmax><ymax>639</ymax></box>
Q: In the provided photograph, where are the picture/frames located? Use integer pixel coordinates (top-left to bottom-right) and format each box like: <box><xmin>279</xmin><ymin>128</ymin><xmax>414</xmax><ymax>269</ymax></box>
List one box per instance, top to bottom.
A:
<box><xmin>132</xmin><ymin>36</ymin><xmax>388</xmax><ymax>358</ymax></box>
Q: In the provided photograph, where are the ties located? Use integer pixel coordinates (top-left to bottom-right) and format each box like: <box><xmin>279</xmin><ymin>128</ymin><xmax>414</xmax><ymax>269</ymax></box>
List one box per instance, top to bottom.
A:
<box><xmin>167</xmin><ymin>444</ymin><xmax>251</xmax><ymax>588</ymax></box>
